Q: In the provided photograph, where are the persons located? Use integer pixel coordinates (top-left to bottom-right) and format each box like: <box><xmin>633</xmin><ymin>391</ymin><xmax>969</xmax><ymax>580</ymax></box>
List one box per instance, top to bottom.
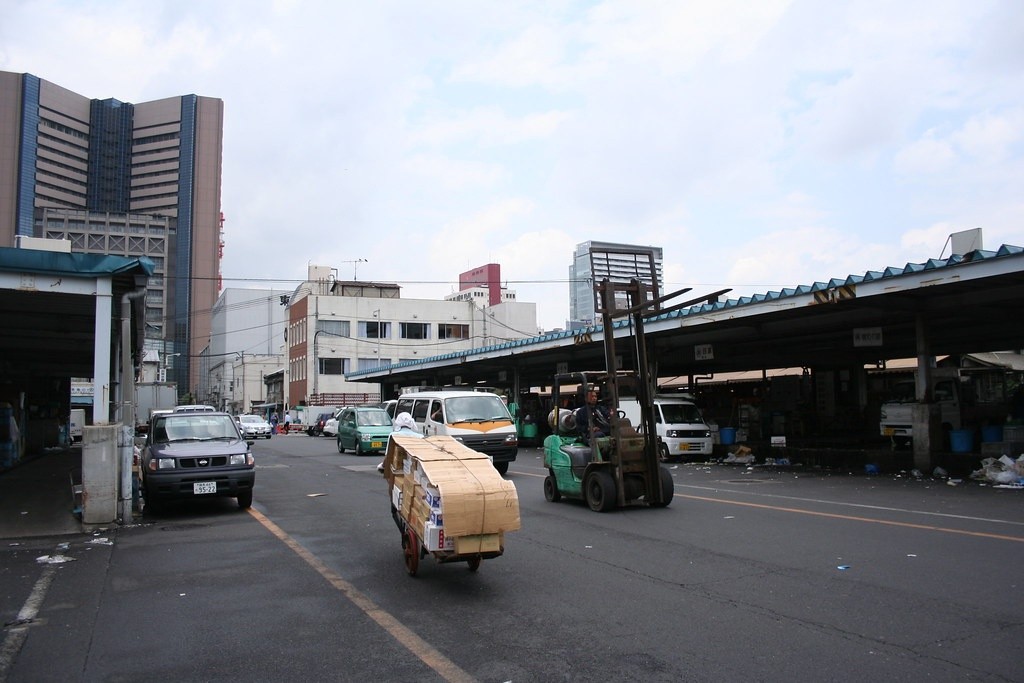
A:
<box><xmin>576</xmin><ymin>390</ymin><xmax>620</xmax><ymax>461</ymax></box>
<box><xmin>431</xmin><ymin>403</ymin><xmax>456</xmax><ymax>422</ymax></box>
<box><xmin>283</xmin><ymin>412</ymin><xmax>290</xmax><ymax>434</ymax></box>
<box><xmin>377</xmin><ymin>412</ymin><xmax>424</xmax><ymax>471</ymax></box>
<box><xmin>270</xmin><ymin>413</ymin><xmax>279</xmax><ymax>435</ymax></box>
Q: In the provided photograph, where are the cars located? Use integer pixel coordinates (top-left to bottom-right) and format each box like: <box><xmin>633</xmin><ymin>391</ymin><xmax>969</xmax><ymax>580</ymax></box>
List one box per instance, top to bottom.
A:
<box><xmin>145</xmin><ymin>403</ymin><xmax>247</xmax><ymax>439</ymax></box>
<box><xmin>139</xmin><ymin>410</ymin><xmax>256</xmax><ymax>514</ymax></box>
<box><xmin>233</xmin><ymin>414</ymin><xmax>272</xmax><ymax>439</ymax></box>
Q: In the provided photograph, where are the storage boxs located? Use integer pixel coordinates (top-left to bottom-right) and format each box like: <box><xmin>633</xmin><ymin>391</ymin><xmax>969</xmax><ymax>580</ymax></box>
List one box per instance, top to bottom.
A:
<box><xmin>1003</xmin><ymin>426</ymin><xmax>1024</xmax><ymax>442</ymax></box>
<box><xmin>981</xmin><ymin>440</ymin><xmax>1016</xmax><ymax>455</ymax></box>
<box><xmin>390</xmin><ymin>445</ymin><xmax>500</xmax><ymax>554</ymax></box>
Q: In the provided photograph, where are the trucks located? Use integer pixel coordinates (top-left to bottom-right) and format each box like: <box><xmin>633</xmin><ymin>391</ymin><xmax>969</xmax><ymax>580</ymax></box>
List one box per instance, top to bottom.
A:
<box><xmin>70</xmin><ymin>408</ymin><xmax>85</xmax><ymax>445</ymax></box>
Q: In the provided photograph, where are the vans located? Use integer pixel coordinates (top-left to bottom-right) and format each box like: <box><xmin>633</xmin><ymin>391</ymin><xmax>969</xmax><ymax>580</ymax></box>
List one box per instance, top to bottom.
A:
<box><xmin>391</xmin><ymin>389</ymin><xmax>518</xmax><ymax>476</ymax></box>
<box><xmin>618</xmin><ymin>393</ymin><xmax>715</xmax><ymax>464</ymax></box>
<box><xmin>324</xmin><ymin>397</ymin><xmax>418</xmax><ymax>455</ymax></box>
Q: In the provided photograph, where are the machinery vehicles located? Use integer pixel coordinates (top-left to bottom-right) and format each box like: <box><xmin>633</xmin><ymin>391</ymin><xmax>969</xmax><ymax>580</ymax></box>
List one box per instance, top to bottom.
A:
<box><xmin>539</xmin><ymin>279</ymin><xmax>734</xmax><ymax>512</ymax></box>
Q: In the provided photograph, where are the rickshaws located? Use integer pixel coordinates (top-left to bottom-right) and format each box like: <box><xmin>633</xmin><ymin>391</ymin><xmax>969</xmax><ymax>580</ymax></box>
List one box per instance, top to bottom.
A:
<box><xmin>378</xmin><ymin>467</ymin><xmax>506</xmax><ymax>575</ymax></box>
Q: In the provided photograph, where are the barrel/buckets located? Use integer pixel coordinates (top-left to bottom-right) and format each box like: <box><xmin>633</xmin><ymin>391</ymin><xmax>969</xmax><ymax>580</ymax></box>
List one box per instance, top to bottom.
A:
<box><xmin>523</xmin><ymin>424</ymin><xmax>538</xmax><ymax>437</ymax></box>
<box><xmin>981</xmin><ymin>426</ymin><xmax>1003</xmax><ymax>443</ymax></box>
<box><xmin>719</xmin><ymin>428</ymin><xmax>738</xmax><ymax>445</ymax></box>
<box><xmin>948</xmin><ymin>430</ymin><xmax>976</xmax><ymax>453</ymax></box>
